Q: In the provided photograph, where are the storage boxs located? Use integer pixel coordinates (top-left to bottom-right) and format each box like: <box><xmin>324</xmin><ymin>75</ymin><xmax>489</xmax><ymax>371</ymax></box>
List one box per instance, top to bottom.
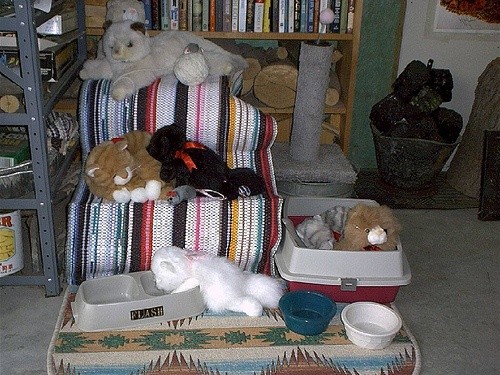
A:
<box><xmin>274</xmin><ymin>194</ymin><xmax>412</xmax><ymax>303</ymax></box>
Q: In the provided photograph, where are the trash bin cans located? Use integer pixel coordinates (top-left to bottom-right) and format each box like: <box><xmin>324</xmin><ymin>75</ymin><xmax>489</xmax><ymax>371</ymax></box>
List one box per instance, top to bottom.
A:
<box><xmin>370</xmin><ymin>121</ymin><xmax>463</xmax><ymax>199</ymax></box>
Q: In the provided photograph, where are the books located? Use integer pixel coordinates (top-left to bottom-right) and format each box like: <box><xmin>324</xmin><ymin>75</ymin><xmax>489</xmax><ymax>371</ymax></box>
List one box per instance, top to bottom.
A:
<box><xmin>144</xmin><ymin>0</ymin><xmax>152</xmax><ymax>29</ymax></box>
<box><xmin>151</xmin><ymin>0</ymin><xmax>161</xmax><ymax>30</ymax></box>
<box><xmin>160</xmin><ymin>0</ymin><xmax>170</xmax><ymax>30</ymax></box>
<box><xmin>170</xmin><ymin>0</ymin><xmax>179</xmax><ymax>30</ymax></box>
<box><xmin>179</xmin><ymin>0</ymin><xmax>188</xmax><ymax>30</ymax></box>
<box><xmin>187</xmin><ymin>0</ymin><xmax>192</xmax><ymax>31</ymax></box>
<box><xmin>192</xmin><ymin>0</ymin><xmax>202</xmax><ymax>31</ymax></box>
<box><xmin>202</xmin><ymin>0</ymin><xmax>209</xmax><ymax>31</ymax></box>
<box><xmin>209</xmin><ymin>0</ymin><xmax>216</xmax><ymax>31</ymax></box>
<box><xmin>215</xmin><ymin>0</ymin><xmax>223</xmax><ymax>31</ymax></box>
<box><xmin>223</xmin><ymin>0</ymin><xmax>232</xmax><ymax>32</ymax></box>
<box><xmin>232</xmin><ymin>0</ymin><xmax>239</xmax><ymax>32</ymax></box>
<box><xmin>239</xmin><ymin>0</ymin><xmax>247</xmax><ymax>32</ymax></box>
<box><xmin>247</xmin><ymin>0</ymin><xmax>254</xmax><ymax>32</ymax></box>
<box><xmin>263</xmin><ymin>0</ymin><xmax>271</xmax><ymax>32</ymax></box>
<box><xmin>270</xmin><ymin>0</ymin><xmax>279</xmax><ymax>32</ymax></box>
<box><xmin>279</xmin><ymin>0</ymin><xmax>285</xmax><ymax>33</ymax></box>
<box><xmin>284</xmin><ymin>0</ymin><xmax>289</xmax><ymax>32</ymax></box>
<box><xmin>288</xmin><ymin>0</ymin><xmax>294</xmax><ymax>33</ymax></box>
<box><xmin>294</xmin><ymin>0</ymin><xmax>301</xmax><ymax>32</ymax></box>
<box><xmin>300</xmin><ymin>0</ymin><xmax>308</xmax><ymax>32</ymax></box>
<box><xmin>307</xmin><ymin>0</ymin><xmax>314</xmax><ymax>33</ymax></box>
<box><xmin>313</xmin><ymin>0</ymin><xmax>320</xmax><ymax>33</ymax></box>
<box><xmin>318</xmin><ymin>0</ymin><xmax>327</xmax><ymax>33</ymax></box>
<box><xmin>329</xmin><ymin>0</ymin><xmax>341</xmax><ymax>33</ymax></box>
<box><xmin>340</xmin><ymin>0</ymin><xmax>348</xmax><ymax>33</ymax></box>
<box><xmin>346</xmin><ymin>0</ymin><xmax>355</xmax><ymax>34</ymax></box>
<box><xmin>254</xmin><ymin>2</ymin><xmax>265</xmax><ymax>32</ymax></box>
<box><xmin>0</xmin><ymin>126</ymin><xmax>30</xmax><ymax>169</ymax></box>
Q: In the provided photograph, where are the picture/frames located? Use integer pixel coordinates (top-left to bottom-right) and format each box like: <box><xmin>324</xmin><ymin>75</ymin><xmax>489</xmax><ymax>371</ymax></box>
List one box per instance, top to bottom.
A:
<box><xmin>422</xmin><ymin>0</ymin><xmax>499</xmax><ymax>39</ymax></box>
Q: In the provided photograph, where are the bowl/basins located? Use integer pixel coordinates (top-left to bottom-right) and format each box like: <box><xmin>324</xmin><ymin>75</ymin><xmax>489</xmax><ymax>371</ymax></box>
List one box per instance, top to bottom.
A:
<box><xmin>278</xmin><ymin>290</ymin><xmax>337</xmax><ymax>336</ymax></box>
<box><xmin>340</xmin><ymin>302</ymin><xmax>403</xmax><ymax>351</ymax></box>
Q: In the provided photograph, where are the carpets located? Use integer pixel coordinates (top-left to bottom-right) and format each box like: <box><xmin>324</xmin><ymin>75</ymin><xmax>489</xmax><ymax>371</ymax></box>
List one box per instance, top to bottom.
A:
<box><xmin>46</xmin><ymin>284</ymin><xmax>422</xmax><ymax>372</ymax></box>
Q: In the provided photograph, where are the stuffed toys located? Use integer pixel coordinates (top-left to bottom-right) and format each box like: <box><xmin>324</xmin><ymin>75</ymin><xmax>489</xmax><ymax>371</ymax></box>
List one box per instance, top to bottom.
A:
<box><xmin>79</xmin><ymin>0</ymin><xmax>146</xmax><ymax>80</ymax></box>
<box><xmin>84</xmin><ymin>18</ymin><xmax>249</xmax><ymax>101</ymax></box>
<box><xmin>146</xmin><ymin>123</ymin><xmax>266</xmax><ymax>199</ymax></box>
<box><xmin>85</xmin><ymin>130</ymin><xmax>174</xmax><ymax>203</ymax></box>
<box><xmin>333</xmin><ymin>204</ymin><xmax>402</xmax><ymax>251</ymax></box>
<box><xmin>321</xmin><ymin>206</ymin><xmax>353</xmax><ymax>240</ymax></box>
<box><xmin>295</xmin><ymin>215</ymin><xmax>336</xmax><ymax>250</ymax></box>
<box><xmin>151</xmin><ymin>247</ymin><xmax>288</xmax><ymax>316</ymax></box>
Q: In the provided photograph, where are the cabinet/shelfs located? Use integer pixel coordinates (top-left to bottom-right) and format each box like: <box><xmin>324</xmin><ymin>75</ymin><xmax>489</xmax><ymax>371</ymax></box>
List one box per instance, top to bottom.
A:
<box><xmin>0</xmin><ymin>0</ymin><xmax>87</xmax><ymax>299</ymax></box>
<box><xmin>56</xmin><ymin>0</ymin><xmax>365</xmax><ymax>159</ymax></box>
